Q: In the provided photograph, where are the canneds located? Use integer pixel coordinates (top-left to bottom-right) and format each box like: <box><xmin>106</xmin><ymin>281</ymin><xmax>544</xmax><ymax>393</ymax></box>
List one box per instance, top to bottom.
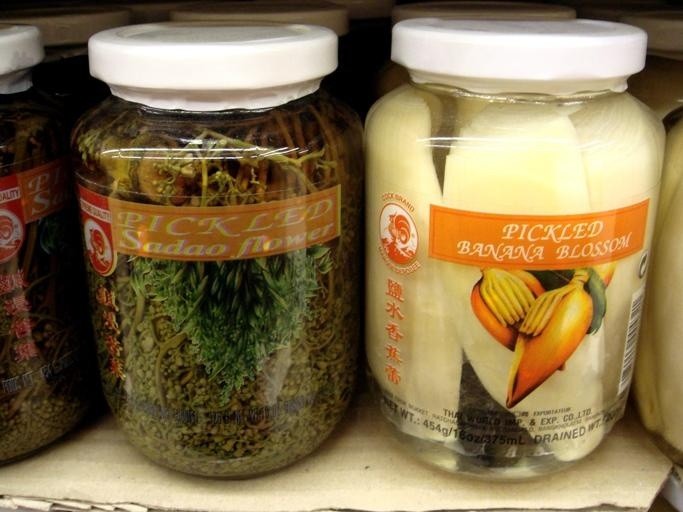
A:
<box><xmin>0</xmin><ymin>0</ymin><xmax>683</xmax><ymax>479</ymax></box>
